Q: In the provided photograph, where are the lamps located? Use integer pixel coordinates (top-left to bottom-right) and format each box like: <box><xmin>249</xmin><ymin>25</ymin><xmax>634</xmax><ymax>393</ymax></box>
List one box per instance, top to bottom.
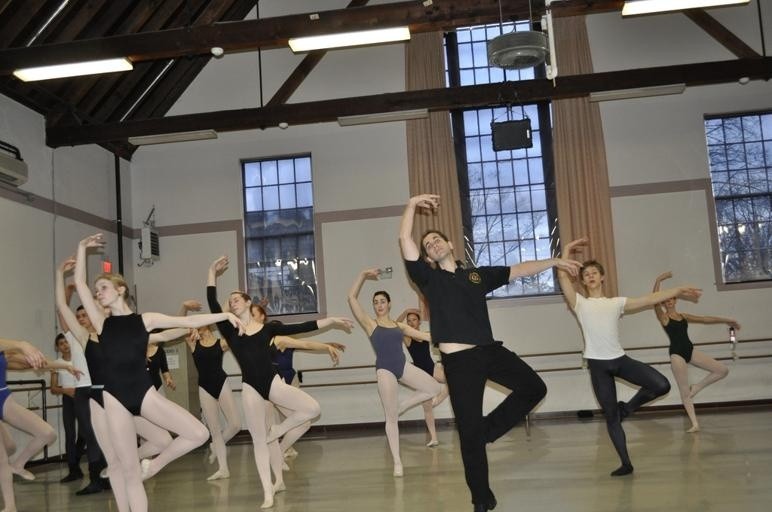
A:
<box><xmin>486</xmin><ymin>0</ymin><xmax>548</xmax><ymax>72</ymax></box>
<box><xmin>622</xmin><ymin>0</ymin><xmax>758</xmax><ymax>18</ymax></box>
<box><xmin>287</xmin><ymin>23</ymin><xmax>411</xmax><ymax>54</ymax></box>
<box><xmin>13</xmin><ymin>51</ymin><xmax>135</xmax><ymax>87</ymax></box>
<box><xmin>586</xmin><ymin>82</ymin><xmax>689</xmax><ymax>103</ymax></box>
<box><xmin>334</xmin><ymin>105</ymin><xmax>430</xmax><ymax>126</ymax></box>
<box><xmin>126</xmin><ymin>129</ymin><xmax>219</xmax><ymax>146</ymax></box>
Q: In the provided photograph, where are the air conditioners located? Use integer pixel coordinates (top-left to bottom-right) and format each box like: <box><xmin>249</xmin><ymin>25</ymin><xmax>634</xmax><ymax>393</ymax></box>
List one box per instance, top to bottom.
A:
<box><xmin>0</xmin><ymin>151</ymin><xmax>29</xmax><ymax>189</ymax></box>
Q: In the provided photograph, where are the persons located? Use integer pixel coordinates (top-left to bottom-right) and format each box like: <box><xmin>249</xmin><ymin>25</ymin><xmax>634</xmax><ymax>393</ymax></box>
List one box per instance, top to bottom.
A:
<box><xmin>396</xmin><ymin>193</ymin><xmax>579</xmax><ymax>512</ymax></box>
<box><xmin>1</xmin><ymin>233</ymin><xmax>358</xmax><ymax>511</ymax></box>
<box><xmin>556</xmin><ymin>234</ymin><xmax>704</xmax><ymax>477</ymax></box>
<box><xmin>346</xmin><ymin>267</ymin><xmax>443</xmax><ymax>479</ymax></box>
<box><xmin>652</xmin><ymin>269</ymin><xmax>743</xmax><ymax>434</ymax></box>
<box><xmin>396</xmin><ymin>307</ymin><xmax>450</xmax><ymax>449</ymax></box>
